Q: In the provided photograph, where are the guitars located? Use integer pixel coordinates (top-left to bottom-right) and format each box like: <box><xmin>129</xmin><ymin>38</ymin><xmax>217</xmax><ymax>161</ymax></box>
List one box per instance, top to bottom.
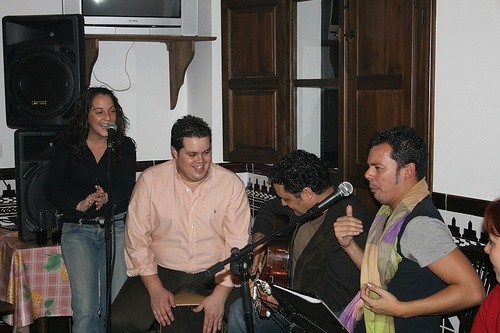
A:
<box><xmin>253</xmin><ymin>240</ymin><xmax>290</xmax><ymax>319</ymax></box>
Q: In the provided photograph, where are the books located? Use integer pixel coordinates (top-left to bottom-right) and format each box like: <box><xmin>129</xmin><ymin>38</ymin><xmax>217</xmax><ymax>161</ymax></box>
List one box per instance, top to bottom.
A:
<box><xmin>269</xmin><ymin>282</ymin><xmax>350</xmax><ymax>333</ymax></box>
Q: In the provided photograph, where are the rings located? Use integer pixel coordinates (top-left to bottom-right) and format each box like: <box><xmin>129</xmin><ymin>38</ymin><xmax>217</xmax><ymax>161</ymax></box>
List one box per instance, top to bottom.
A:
<box><xmin>88</xmin><ymin>201</ymin><xmax>92</xmax><ymax>204</ymax></box>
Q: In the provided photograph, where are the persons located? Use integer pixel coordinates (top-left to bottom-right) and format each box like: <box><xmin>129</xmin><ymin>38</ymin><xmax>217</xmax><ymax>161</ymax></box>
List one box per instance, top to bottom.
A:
<box><xmin>44</xmin><ymin>86</ymin><xmax>136</xmax><ymax>333</ymax></box>
<box><xmin>110</xmin><ymin>114</ymin><xmax>251</xmax><ymax>333</ymax></box>
<box><xmin>334</xmin><ymin>125</ymin><xmax>484</xmax><ymax>333</ymax></box>
<box><xmin>228</xmin><ymin>150</ymin><xmax>376</xmax><ymax>333</ymax></box>
<box><xmin>469</xmin><ymin>198</ymin><xmax>500</xmax><ymax>333</ymax></box>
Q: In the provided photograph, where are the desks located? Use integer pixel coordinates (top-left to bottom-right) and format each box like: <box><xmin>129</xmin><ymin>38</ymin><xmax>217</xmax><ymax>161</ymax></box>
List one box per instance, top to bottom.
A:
<box><xmin>0</xmin><ymin>226</ymin><xmax>101</xmax><ymax>333</ymax></box>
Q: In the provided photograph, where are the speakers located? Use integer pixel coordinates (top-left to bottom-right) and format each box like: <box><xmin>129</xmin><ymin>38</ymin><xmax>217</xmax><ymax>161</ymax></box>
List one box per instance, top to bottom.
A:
<box><xmin>2</xmin><ymin>14</ymin><xmax>86</xmax><ymax>129</ymax></box>
<box><xmin>14</xmin><ymin>129</ymin><xmax>64</xmax><ymax>241</ymax></box>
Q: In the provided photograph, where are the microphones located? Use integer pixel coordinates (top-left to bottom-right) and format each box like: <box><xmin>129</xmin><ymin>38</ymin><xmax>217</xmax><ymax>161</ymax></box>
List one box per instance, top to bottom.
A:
<box><xmin>107</xmin><ymin>122</ymin><xmax>117</xmax><ymax>149</ymax></box>
<box><xmin>307</xmin><ymin>181</ymin><xmax>354</xmax><ymax>215</ymax></box>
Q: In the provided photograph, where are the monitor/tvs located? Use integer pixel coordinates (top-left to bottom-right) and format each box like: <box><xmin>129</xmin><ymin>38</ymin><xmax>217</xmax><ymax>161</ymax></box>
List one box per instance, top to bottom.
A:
<box><xmin>62</xmin><ymin>0</ymin><xmax>199</xmax><ymax>36</ymax></box>
